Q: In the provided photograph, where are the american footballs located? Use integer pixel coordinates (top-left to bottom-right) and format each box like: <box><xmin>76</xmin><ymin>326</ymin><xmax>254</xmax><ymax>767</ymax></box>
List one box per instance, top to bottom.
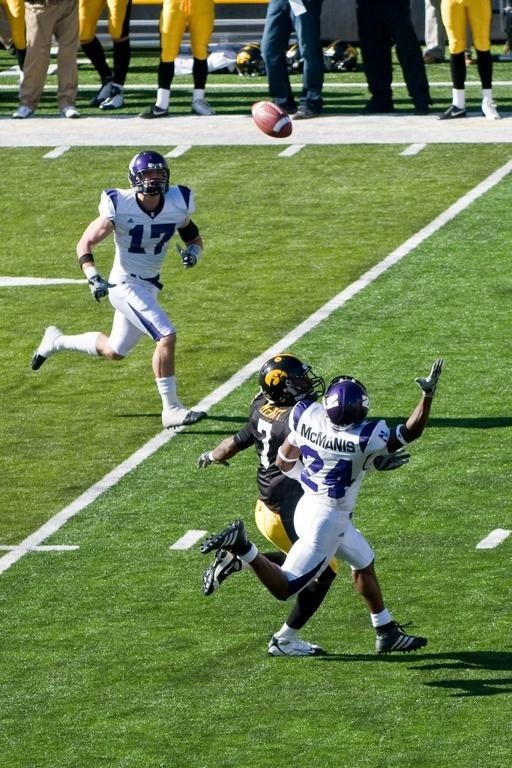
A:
<box><xmin>251</xmin><ymin>101</ymin><xmax>291</xmax><ymax>137</ymax></box>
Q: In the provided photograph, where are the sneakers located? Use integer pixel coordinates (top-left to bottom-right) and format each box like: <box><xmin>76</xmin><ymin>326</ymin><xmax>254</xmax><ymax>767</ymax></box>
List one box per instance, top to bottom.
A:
<box><xmin>423</xmin><ymin>52</ymin><xmax>441</xmax><ymax>63</ymax></box>
<box><xmin>465</xmin><ymin>54</ymin><xmax>475</xmax><ymax>64</ymax></box>
<box><xmin>10</xmin><ymin>82</ymin><xmax>213</xmax><ymax>119</ymax></box>
<box><xmin>482</xmin><ymin>98</ymin><xmax>500</xmax><ymax>118</ymax></box>
<box><xmin>293</xmin><ymin>104</ymin><xmax>322</xmax><ymax>119</ymax></box>
<box><xmin>437</xmin><ymin>105</ymin><xmax>466</xmax><ymax>119</ymax></box>
<box><xmin>32</xmin><ymin>326</ymin><xmax>62</xmax><ymax>370</ymax></box>
<box><xmin>162</xmin><ymin>408</ymin><xmax>205</xmax><ymax>429</ymax></box>
<box><xmin>197</xmin><ymin>517</ymin><xmax>244</xmax><ymax>593</ymax></box>
<box><xmin>376</xmin><ymin>620</ymin><xmax>426</xmax><ymax>653</ymax></box>
<box><xmin>268</xmin><ymin>633</ymin><xmax>322</xmax><ymax>656</ymax></box>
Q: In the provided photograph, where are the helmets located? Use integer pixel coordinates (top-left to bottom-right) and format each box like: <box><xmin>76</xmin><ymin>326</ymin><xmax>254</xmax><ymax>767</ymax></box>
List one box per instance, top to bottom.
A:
<box><xmin>236</xmin><ymin>42</ymin><xmax>360</xmax><ymax>75</ymax></box>
<box><xmin>128</xmin><ymin>151</ymin><xmax>169</xmax><ymax>195</ymax></box>
<box><xmin>259</xmin><ymin>355</ymin><xmax>325</xmax><ymax>407</ymax></box>
<box><xmin>324</xmin><ymin>375</ymin><xmax>370</xmax><ymax>431</ymax></box>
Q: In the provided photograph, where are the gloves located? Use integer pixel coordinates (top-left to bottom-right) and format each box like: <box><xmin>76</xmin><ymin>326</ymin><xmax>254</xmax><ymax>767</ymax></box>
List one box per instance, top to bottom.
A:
<box><xmin>177</xmin><ymin>245</ymin><xmax>196</xmax><ymax>268</ymax></box>
<box><xmin>89</xmin><ymin>275</ymin><xmax>116</xmax><ymax>303</ymax></box>
<box><xmin>415</xmin><ymin>358</ymin><xmax>442</xmax><ymax>396</ymax></box>
<box><xmin>378</xmin><ymin>448</ymin><xmax>409</xmax><ymax>470</ymax></box>
<box><xmin>197</xmin><ymin>451</ymin><xmax>229</xmax><ymax>468</ymax></box>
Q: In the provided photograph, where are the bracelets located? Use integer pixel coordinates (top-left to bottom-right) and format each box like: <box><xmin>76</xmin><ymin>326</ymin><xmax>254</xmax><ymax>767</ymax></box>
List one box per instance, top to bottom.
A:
<box><xmin>78</xmin><ymin>253</ymin><xmax>95</xmax><ymax>268</ymax></box>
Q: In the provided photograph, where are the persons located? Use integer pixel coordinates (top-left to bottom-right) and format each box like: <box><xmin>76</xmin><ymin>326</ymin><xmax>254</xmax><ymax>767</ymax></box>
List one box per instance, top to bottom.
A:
<box><xmin>0</xmin><ymin>0</ymin><xmax>25</xmax><ymax>70</ymax></box>
<box><xmin>12</xmin><ymin>0</ymin><xmax>81</xmax><ymax>118</ymax></box>
<box><xmin>79</xmin><ymin>0</ymin><xmax>132</xmax><ymax>110</ymax></box>
<box><xmin>139</xmin><ymin>0</ymin><xmax>216</xmax><ymax>118</ymax></box>
<box><xmin>260</xmin><ymin>0</ymin><xmax>324</xmax><ymax>120</ymax></box>
<box><xmin>356</xmin><ymin>0</ymin><xmax>430</xmax><ymax>115</ymax></box>
<box><xmin>423</xmin><ymin>0</ymin><xmax>476</xmax><ymax>64</ymax></box>
<box><xmin>436</xmin><ymin>0</ymin><xmax>501</xmax><ymax>120</ymax></box>
<box><xmin>33</xmin><ymin>149</ymin><xmax>208</xmax><ymax>427</ymax></box>
<box><xmin>196</xmin><ymin>355</ymin><xmax>338</xmax><ymax>656</ymax></box>
<box><xmin>199</xmin><ymin>357</ymin><xmax>445</xmax><ymax>652</ymax></box>
